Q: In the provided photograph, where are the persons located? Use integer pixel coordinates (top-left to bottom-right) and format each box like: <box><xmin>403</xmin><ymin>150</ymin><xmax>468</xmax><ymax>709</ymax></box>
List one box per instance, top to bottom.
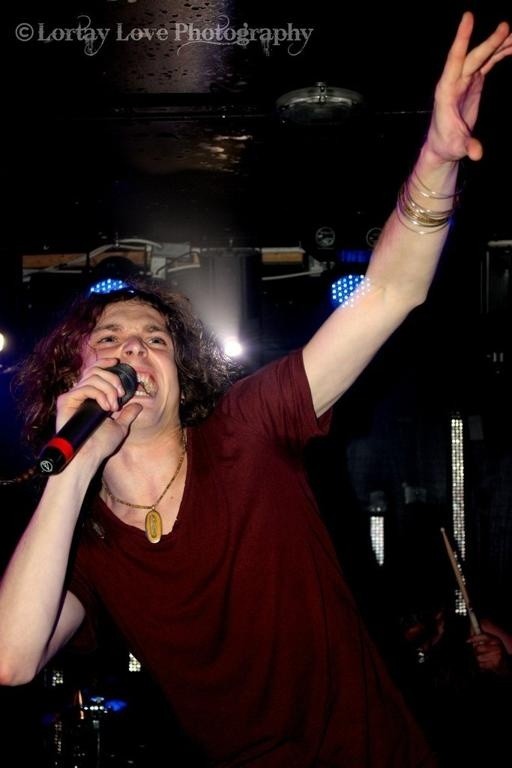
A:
<box><xmin>0</xmin><ymin>8</ymin><xmax>511</xmax><ymax>768</ymax></box>
<box><xmin>390</xmin><ymin>603</ymin><xmax>512</xmax><ymax>768</ymax></box>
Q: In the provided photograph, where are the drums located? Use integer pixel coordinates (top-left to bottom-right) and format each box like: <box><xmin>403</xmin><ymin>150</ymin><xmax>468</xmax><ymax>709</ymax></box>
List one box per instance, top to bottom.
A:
<box><xmin>39</xmin><ymin>665</ymin><xmax>149</xmax><ymax>765</ymax></box>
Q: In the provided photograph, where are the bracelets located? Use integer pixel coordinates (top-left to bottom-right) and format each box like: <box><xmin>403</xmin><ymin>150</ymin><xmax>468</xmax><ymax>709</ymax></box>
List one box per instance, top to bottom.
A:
<box><xmin>394</xmin><ymin>168</ymin><xmax>464</xmax><ymax>238</ymax></box>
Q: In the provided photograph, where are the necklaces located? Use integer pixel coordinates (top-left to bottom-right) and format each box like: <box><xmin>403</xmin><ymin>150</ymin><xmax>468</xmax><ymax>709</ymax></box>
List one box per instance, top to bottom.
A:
<box><xmin>99</xmin><ymin>427</ymin><xmax>190</xmax><ymax>546</ymax></box>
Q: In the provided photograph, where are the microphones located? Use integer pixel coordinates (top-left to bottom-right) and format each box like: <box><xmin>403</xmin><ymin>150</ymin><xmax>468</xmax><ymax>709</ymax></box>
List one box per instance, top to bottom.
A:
<box><xmin>40</xmin><ymin>362</ymin><xmax>137</xmax><ymax>476</ymax></box>
